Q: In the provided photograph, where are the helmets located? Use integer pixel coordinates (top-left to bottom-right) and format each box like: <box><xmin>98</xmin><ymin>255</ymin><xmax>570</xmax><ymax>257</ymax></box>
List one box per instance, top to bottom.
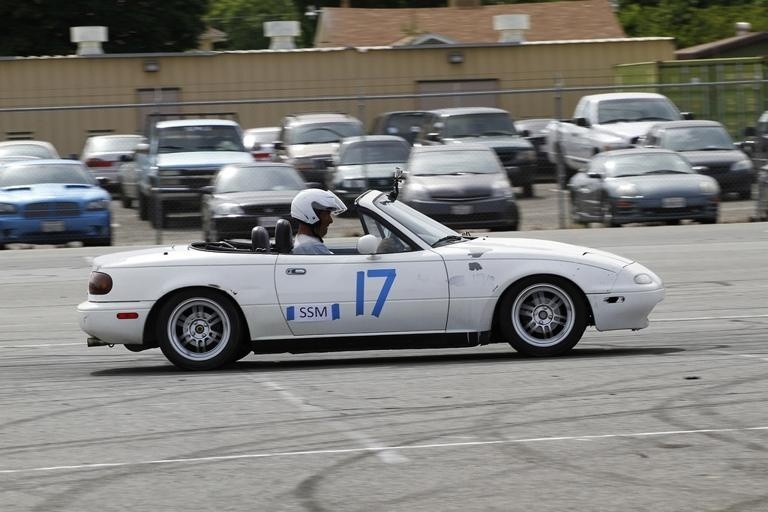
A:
<box><xmin>291</xmin><ymin>188</ymin><xmax>347</xmax><ymax>225</ymax></box>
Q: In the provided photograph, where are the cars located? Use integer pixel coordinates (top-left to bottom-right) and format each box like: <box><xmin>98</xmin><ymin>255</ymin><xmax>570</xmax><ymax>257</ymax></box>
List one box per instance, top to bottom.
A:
<box><xmin>242</xmin><ymin>111</ymin><xmax>365</xmax><ymax>185</ymax></box>
<box><xmin>511</xmin><ymin>118</ymin><xmax>561</xmax><ymax>182</ymax></box>
<box><xmin>639</xmin><ymin>119</ymin><xmax>757</xmax><ymax>199</ymax></box>
<box><xmin>69</xmin><ymin>133</ymin><xmax>148</xmax><ymax>193</ymax></box>
<box><xmin>318</xmin><ymin>135</ymin><xmax>410</xmax><ymax>216</ymax></box>
<box><xmin>114</xmin><ymin>139</ymin><xmax>150</xmax><ymax>209</ymax></box>
<box><xmin>0</xmin><ymin>140</ymin><xmax>61</xmax><ymax>169</ymax></box>
<box><xmin>394</xmin><ymin>145</ymin><xmax>523</xmax><ymax>232</ymax></box>
<box><xmin>568</xmin><ymin>147</ymin><xmax>722</xmax><ymax>228</ymax></box>
<box><xmin>0</xmin><ymin>158</ymin><xmax>113</xmax><ymax>246</ymax></box>
<box><xmin>200</xmin><ymin>160</ymin><xmax>321</xmax><ymax>243</ymax></box>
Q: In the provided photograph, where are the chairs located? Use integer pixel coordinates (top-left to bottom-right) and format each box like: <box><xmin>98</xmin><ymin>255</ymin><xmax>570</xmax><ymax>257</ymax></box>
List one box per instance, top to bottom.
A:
<box><xmin>250</xmin><ymin>220</ymin><xmax>295</xmax><ymax>254</ymax></box>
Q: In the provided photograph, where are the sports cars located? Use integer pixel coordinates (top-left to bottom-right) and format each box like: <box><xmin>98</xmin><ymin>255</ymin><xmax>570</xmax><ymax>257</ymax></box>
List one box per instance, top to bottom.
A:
<box><xmin>74</xmin><ymin>191</ymin><xmax>664</xmax><ymax>366</ymax></box>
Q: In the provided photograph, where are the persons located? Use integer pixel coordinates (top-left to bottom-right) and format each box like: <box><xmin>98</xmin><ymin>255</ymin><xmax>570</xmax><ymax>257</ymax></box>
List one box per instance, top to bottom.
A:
<box><xmin>288</xmin><ymin>186</ymin><xmax>347</xmax><ymax>254</ymax></box>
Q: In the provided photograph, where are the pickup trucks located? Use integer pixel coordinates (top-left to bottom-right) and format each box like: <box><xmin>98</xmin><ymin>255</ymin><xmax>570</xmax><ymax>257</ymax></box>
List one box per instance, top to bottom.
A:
<box><xmin>544</xmin><ymin>91</ymin><xmax>695</xmax><ymax>190</ymax></box>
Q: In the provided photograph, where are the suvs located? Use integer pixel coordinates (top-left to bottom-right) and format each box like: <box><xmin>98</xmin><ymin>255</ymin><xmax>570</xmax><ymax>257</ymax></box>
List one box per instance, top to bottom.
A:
<box><xmin>410</xmin><ymin>106</ymin><xmax>538</xmax><ymax>198</ymax></box>
<box><xmin>366</xmin><ymin>109</ymin><xmax>434</xmax><ymax>145</ymax></box>
<box><xmin>133</xmin><ymin>117</ymin><xmax>257</xmax><ymax>230</ymax></box>
